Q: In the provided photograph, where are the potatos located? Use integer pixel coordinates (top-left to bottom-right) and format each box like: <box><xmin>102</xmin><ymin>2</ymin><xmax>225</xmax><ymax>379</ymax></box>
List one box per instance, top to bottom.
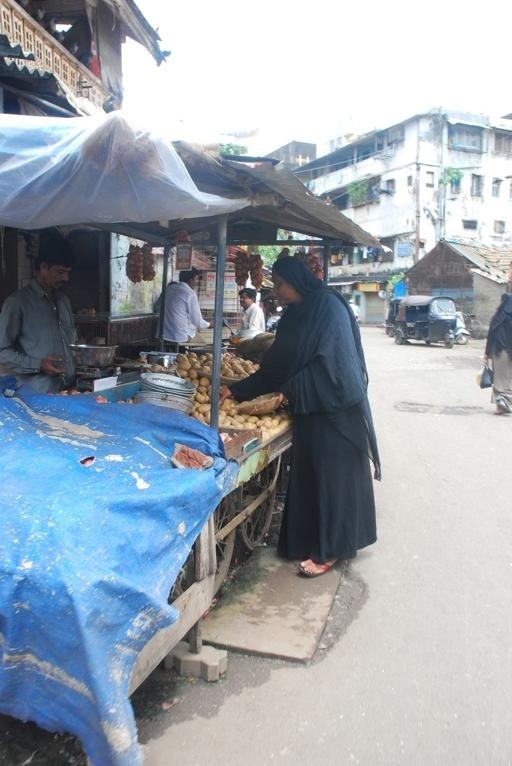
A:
<box><xmin>136</xmin><ymin>352</ymin><xmax>291</xmax><ymax>441</ymax></box>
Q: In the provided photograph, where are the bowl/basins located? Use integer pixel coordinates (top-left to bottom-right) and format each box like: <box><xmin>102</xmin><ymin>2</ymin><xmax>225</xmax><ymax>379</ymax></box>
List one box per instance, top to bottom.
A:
<box><xmin>67</xmin><ymin>343</ymin><xmax>120</xmax><ymax>368</ymax></box>
<box><xmin>132</xmin><ymin>371</ymin><xmax>196</xmax><ymax>414</ymax></box>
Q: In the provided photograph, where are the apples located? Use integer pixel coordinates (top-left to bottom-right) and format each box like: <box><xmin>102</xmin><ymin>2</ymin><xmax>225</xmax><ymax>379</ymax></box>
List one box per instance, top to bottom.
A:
<box><xmin>126</xmin><ymin>243</ymin><xmax>155</xmax><ymax>282</ymax></box>
<box><xmin>234</xmin><ymin>250</ymin><xmax>263</xmax><ymax>289</ymax></box>
<box><xmin>294</xmin><ymin>251</ymin><xmax>323</xmax><ymax>280</ymax></box>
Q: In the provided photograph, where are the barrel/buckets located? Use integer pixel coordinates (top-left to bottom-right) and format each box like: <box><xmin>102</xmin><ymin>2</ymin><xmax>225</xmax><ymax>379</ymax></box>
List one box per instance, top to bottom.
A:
<box><xmin>133</xmin><ymin>372</ymin><xmax>195</xmax><ymax>416</ymax></box>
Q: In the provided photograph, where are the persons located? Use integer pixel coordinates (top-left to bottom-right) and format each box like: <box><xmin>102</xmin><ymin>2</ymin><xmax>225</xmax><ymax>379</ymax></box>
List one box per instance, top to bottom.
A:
<box><xmin>0</xmin><ymin>241</ymin><xmax>79</xmax><ymax>394</ymax></box>
<box><xmin>219</xmin><ymin>255</ymin><xmax>381</xmax><ymax>578</ymax></box>
<box><xmin>154</xmin><ymin>270</ymin><xmax>215</xmax><ymax>353</ymax></box>
<box><xmin>230</xmin><ymin>288</ymin><xmax>266</xmax><ymax>344</ymax></box>
<box><xmin>484</xmin><ymin>294</ymin><xmax>512</xmax><ymax>414</ymax></box>
<box><xmin>348</xmin><ymin>299</ymin><xmax>360</xmax><ymax>321</ymax></box>
<box><xmin>266</xmin><ymin>304</ymin><xmax>281</xmax><ymax>333</ymax></box>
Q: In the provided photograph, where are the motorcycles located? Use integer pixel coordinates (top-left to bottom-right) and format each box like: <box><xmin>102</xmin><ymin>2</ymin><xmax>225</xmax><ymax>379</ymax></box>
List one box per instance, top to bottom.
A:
<box><xmin>384</xmin><ymin>295</ymin><xmax>457</xmax><ymax>349</ymax></box>
<box><xmin>455</xmin><ymin>305</ymin><xmax>472</xmax><ymax>345</ymax></box>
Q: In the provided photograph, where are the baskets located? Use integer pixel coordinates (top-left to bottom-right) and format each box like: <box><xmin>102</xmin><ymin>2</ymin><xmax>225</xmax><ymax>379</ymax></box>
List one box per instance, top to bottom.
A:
<box><xmin>197</xmin><ymin>370</ymin><xmax>241</xmax><ymax>388</ymax></box>
<box><xmin>237</xmin><ymin>392</ymin><xmax>283</xmax><ymax>415</ymax></box>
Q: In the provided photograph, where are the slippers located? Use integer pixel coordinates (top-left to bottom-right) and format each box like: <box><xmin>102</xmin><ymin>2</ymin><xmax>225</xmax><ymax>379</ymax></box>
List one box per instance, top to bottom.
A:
<box><xmin>496</xmin><ymin>397</ymin><xmax>512</xmax><ymax>413</ymax></box>
<box><xmin>297</xmin><ymin>559</ymin><xmax>336</xmax><ymax>577</ymax></box>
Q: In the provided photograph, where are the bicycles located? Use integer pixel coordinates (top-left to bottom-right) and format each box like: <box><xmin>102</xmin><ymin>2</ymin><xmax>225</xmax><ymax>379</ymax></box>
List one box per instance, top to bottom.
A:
<box><xmin>463</xmin><ymin>314</ymin><xmax>482</xmax><ymax>338</ymax></box>
